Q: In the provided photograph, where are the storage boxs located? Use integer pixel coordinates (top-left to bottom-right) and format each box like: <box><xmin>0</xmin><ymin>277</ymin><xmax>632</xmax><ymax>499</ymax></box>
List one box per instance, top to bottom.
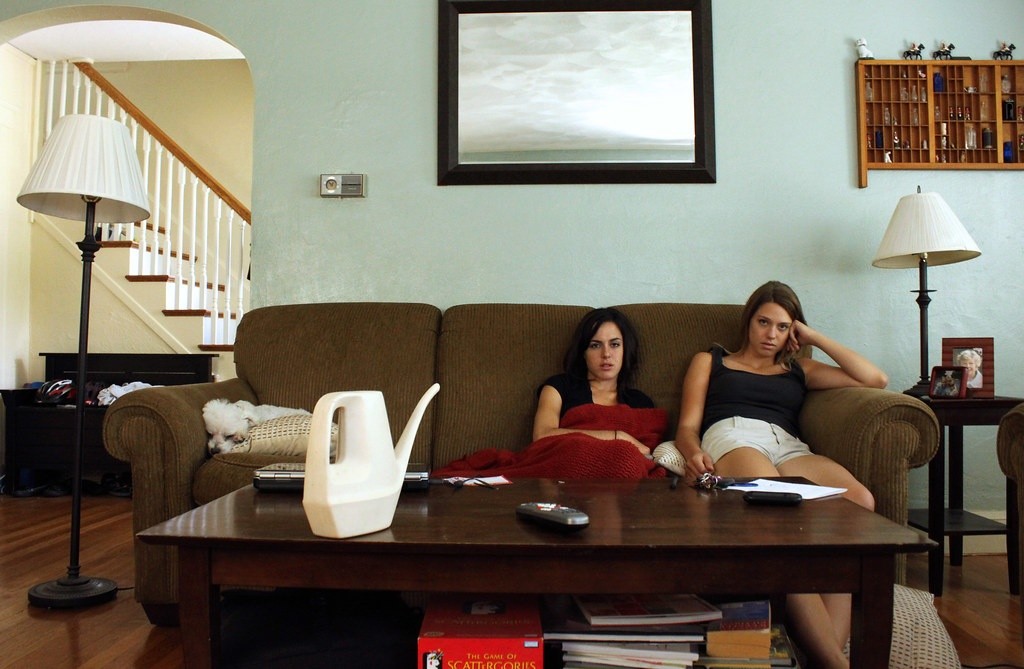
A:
<box><xmin>416</xmin><ymin>598</ymin><xmax>543</xmax><ymax>669</ymax></box>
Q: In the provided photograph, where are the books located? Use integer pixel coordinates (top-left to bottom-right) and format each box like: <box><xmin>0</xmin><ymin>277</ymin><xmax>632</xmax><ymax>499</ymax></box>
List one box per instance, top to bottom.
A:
<box><xmin>543</xmin><ymin>593</ymin><xmax>796</xmax><ymax>669</ymax></box>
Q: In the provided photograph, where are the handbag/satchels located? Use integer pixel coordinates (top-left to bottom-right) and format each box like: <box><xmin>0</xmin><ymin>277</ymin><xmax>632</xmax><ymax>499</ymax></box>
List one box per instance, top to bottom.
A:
<box><xmin>98</xmin><ymin>381</ymin><xmax>165</xmax><ymax>406</ymax></box>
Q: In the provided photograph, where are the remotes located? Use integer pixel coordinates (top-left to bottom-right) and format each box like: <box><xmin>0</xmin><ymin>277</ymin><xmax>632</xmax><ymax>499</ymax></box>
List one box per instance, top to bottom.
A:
<box><xmin>515</xmin><ymin>502</ymin><xmax>590</xmax><ymax>533</ymax></box>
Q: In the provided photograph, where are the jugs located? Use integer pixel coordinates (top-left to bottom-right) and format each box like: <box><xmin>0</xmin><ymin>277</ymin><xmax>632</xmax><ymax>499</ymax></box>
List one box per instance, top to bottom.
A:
<box><xmin>301</xmin><ymin>382</ymin><xmax>441</xmax><ymax>539</ymax></box>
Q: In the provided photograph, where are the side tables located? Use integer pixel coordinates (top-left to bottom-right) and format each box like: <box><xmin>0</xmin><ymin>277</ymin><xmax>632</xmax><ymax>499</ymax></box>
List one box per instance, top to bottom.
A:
<box><xmin>895</xmin><ymin>396</ymin><xmax>1024</xmax><ymax>597</ymax></box>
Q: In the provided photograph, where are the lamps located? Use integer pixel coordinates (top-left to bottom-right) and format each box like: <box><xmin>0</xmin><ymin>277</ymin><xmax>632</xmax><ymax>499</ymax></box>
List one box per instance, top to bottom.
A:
<box><xmin>18</xmin><ymin>114</ymin><xmax>150</xmax><ymax>611</ymax></box>
<box><xmin>871</xmin><ymin>186</ymin><xmax>982</xmax><ymax>398</ymax></box>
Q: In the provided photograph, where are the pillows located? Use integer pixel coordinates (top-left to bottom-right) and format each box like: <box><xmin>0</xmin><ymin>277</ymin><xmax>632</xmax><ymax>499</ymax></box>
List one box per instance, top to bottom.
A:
<box><xmin>228</xmin><ymin>414</ymin><xmax>339</xmax><ymax>460</ymax></box>
<box><xmin>841</xmin><ymin>583</ymin><xmax>961</xmax><ymax>669</ymax></box>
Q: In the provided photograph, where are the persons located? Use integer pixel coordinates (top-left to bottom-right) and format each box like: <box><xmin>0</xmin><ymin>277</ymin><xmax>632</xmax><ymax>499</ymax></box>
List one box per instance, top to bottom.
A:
<box><xmin>673</xmin><ymin>280</ymin><xmax>889</xmax><ymax>669</ymax></box>
<box><xmin>534</xmin><ymin>307</ymin><xmax>654</xmax><ymax>461</ymax></box>
<box><xmin>956</xmin><ymin>350</ymin><xmax>982</xmax><ymax>388</ymax></box>
<box><xmin>934</xmin><ymin>374</ymin><xmax>958</xmax><ymax>396</ymax></box>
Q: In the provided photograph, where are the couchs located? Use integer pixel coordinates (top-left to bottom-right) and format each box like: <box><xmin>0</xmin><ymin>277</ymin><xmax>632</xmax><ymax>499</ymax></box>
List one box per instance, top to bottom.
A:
<box><xmin>102</xmin><ymin>302</ymin><xmax>940</xmax><ymax>590</ymax></box>
<box><xmin>996</xmin><ymin>403</ymin><xmax>1024</xmax><ymax>598</ymax></box>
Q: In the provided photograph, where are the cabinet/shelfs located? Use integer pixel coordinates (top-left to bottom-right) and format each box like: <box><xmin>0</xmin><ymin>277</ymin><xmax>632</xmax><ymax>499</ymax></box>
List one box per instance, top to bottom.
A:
<box><xmin>855</xmin><ymin>57</ymin><xmax>1024</xmax><ymax>188</ymax></box>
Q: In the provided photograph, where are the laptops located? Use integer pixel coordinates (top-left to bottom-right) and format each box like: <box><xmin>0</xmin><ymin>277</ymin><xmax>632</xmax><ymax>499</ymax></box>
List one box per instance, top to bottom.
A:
<box><xmin>254</xmin><ymin>462</ymin><xmax>431</xmax><ymax>493</ymax></box>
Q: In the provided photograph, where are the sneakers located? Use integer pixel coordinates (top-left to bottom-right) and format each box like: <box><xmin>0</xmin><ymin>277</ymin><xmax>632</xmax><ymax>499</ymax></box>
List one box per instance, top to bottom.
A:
<box><xmin>101</xmin><ymin>477</ymin><xmax>130</xmax><ymax>496</ymax></box>
<box><xmin>45</xmin><ymin>478</ymin><xmax>73</xmax><ymax>494</ymax></box>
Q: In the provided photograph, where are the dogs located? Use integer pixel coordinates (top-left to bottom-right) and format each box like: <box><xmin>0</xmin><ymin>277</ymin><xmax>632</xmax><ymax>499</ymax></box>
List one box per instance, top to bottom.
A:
<box><xmin>202</xmin><ymin>398</ymin><xmax>311</xmax><ymax>456</ymax></box>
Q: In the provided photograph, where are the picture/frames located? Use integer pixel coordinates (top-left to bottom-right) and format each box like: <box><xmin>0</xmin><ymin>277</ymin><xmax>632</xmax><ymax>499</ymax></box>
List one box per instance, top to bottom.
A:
<box><xmin>437</xmin><ymin>0</ymin><xmax>717</xmax><ymax>186</ymax></box>
<box><xmin>942</xmin><ymin>336</ymin><xmax>994</xmax><ymax>399</ymax></box>
<box><xmin>929</xmin><ymin>365</ymin><xmax>968</xmax><ymax>399</ymax></box>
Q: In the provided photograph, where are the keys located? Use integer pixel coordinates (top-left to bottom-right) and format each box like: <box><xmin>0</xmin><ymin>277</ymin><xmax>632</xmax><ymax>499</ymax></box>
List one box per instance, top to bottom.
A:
<box><xmin>687</xmin><ymin>472</ymin><xmax>736</xmax><ymax>492</ymax></box>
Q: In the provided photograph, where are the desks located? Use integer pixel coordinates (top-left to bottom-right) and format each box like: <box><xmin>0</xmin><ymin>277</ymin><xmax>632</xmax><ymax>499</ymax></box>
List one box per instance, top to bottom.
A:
<box><xmin>135</xmin><ymin>476</ymin><xmax>938</xmax><ymax>669</ymax></box>
